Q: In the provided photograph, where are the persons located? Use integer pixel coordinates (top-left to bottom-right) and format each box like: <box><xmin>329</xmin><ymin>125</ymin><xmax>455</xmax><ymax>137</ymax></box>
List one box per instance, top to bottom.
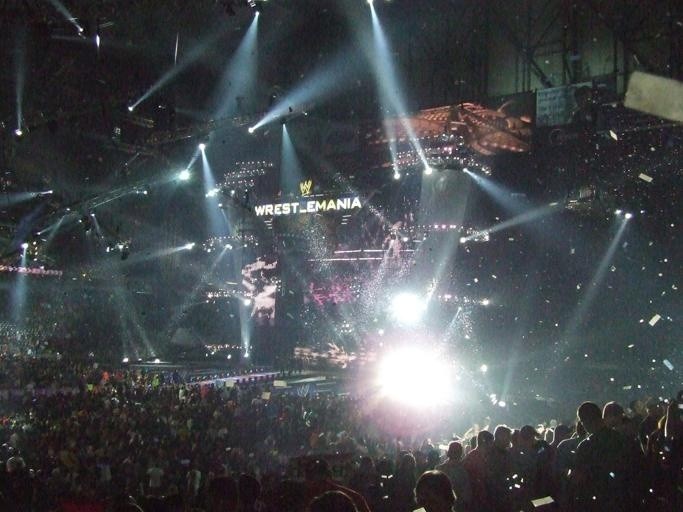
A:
<box><xmin>1</xmin><ymin>294</ymin><xmax>683</xmax><ymax>511</ymax></box>
